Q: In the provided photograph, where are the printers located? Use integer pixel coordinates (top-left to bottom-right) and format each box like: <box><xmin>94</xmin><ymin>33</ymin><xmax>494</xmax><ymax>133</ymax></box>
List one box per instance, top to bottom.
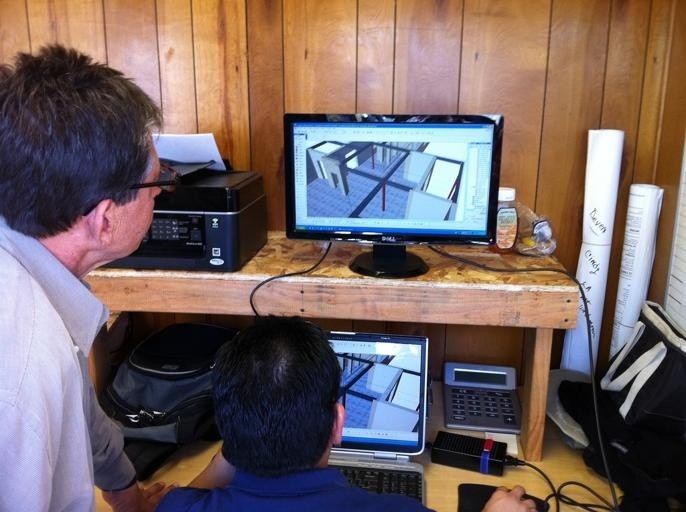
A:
<box><xmin>99</xmin><ymin>171</ymin><xmax>268</xmax><ymax>273</ymax></box>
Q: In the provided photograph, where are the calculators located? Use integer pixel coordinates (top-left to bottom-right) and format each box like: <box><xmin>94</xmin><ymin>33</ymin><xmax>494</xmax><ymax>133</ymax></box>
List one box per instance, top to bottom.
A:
<box><xmin>443</xmin><ymin>362</ymin><xmax>522</xmax><ymax>435</ymax></box>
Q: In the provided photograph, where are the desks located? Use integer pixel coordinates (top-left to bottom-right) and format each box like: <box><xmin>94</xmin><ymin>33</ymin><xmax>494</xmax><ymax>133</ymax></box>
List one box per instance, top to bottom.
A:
<box><xmin>82</xmin><ymin>229</ymin><xmax>581</xmax><ymax>461</ymax></box>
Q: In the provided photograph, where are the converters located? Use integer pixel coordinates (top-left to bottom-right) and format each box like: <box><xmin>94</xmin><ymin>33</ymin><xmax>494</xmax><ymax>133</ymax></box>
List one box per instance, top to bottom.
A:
<box><xmin>431</xmin><ymin>431</ymin><xmax>508</xmax><ymax>477</ymax></box>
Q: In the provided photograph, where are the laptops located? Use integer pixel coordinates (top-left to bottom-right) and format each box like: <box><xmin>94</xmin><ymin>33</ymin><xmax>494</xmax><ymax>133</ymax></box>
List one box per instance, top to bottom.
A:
<box><xmin>324</xmin><ymin>330</ymin><xmax>430</xmax><ymax>507</ymax></box>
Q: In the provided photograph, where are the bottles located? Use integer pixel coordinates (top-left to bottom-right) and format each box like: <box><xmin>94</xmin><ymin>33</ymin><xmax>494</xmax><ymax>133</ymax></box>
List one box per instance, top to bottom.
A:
<box><xmin>489</xmin><ymin>186</ymin><xmax>520</xmax><ymax>253</ymax></box>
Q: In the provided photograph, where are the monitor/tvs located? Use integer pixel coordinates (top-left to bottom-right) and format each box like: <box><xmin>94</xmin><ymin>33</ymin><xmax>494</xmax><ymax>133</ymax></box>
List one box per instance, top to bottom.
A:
<box><xmin>284</xmin><ymin>113</ymin><xmax>504</xmax><ymax>279</ymax></box>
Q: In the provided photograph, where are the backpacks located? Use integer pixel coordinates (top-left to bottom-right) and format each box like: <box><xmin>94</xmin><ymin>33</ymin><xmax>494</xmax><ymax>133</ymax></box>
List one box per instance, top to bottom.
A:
<box><xmin>91</xmin><ymin>320</ymin><xmax>248</xmax><ymax>482</ymax></box>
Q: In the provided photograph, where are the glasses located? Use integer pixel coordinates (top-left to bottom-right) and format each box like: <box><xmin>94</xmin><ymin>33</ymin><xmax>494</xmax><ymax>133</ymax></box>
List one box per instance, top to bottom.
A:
<box><xmin>80</xmin><ymin>160</ymin><xmax>186</xmax><ymax>218</ymax></box>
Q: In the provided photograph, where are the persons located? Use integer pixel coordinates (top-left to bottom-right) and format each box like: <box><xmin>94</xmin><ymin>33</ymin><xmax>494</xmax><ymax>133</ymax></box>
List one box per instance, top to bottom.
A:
<box><xmin>0</xmin><ymin>41</ymin><xmax>181</xmax><ymax>510</ymax></box>
<box><xmin>156</xmin><ymin>315</ymin><xmax>541</xmax><ymax>512</ymax></box>
